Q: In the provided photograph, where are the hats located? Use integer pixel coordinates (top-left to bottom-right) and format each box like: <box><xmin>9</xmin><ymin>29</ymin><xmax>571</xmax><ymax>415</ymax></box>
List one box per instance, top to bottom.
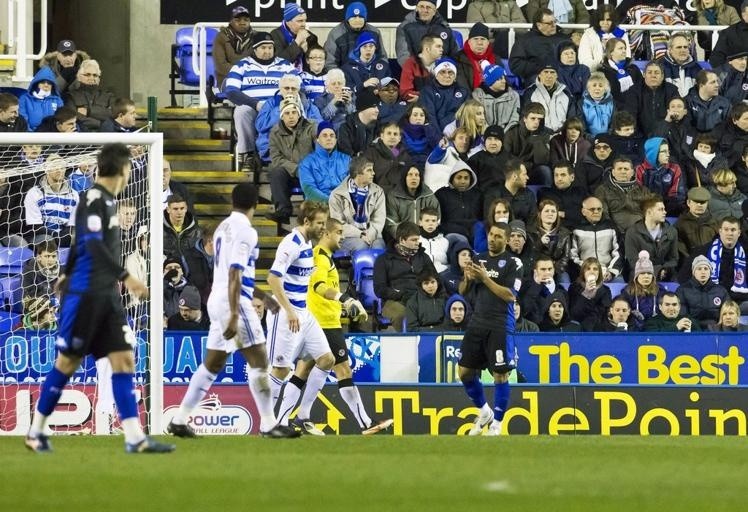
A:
<box><xmin>345</xmin><ymin>2</ymin><xmax>368</xmax><ymax>22</ymax></box>
<box><xmin>283</xmin><ymin>3</ymin><xmax>307</xmax><ymax>22</ymax></box>
<box><xmin>231</xmin><ymin>6</ymin><xmax>251</xmax><ymax>18</ymax></box>
<box><xmin>433</xmin><ymin>22</ymin><xmax>558</xmax><ymax>87</ymax></box>
<box><xmin>252</xmin><ymin>31</ymin><xmax>275</xmax><ymax>49</ymax></box>
<box><xmin>354</xmin><ymin>32</ymin><xmax>377</xmax><ymax>52</ymax></box>
<box><xmin>57</xmin><ymin>39</ymin><xmax>77</xmax><ymax>53</ymax></box>
<box><xmin>726</xmin><ymin>43</ymin><xmax>748</xmax><ymax>61</ymax></box>
<box><xmin>378</xmin><ymin>76</ymin><xmax>400</xmax><ymax>91</ymax></box>
<box><xmin>356</xmin><ymin>90</ymin><xmax>378</xmax><ymax>111</ymax></box>
<box><xmin>279</xmin><ymin>98</ymin><xmax>302</xmax><ymax>120</ymax></box>
<box><xmin>318</xmin><ymin>121</ymin><xmax>337</xmax><ymax>137</ymax></box>
<box><xmin>486</xmin><ymin>123</ymin><xmax>502</xmax><ymax>139</ymax></box>
<box><xmin>46</xmin><ymin>153</ymin><xmax>62</xmax><ymax>160</ymax></box>
<box><xmin>687</xmin><ymin>186</ymin><xmax>713</xmax><ymax>203</ymax></box>
<box><xmin>508</xmin><ymin>219</ymin><xmax>527</xmax><ymax>240</ymax></box>
<box><xmin>163</xmin><ymin>248</ymin><xmax>185</xmax><ymax>276</ymax></box>
<box><xmin>632</xmin><ymin>250</ymin><xmax>657</xmax><ymax>320</ymax></box>
<box><xmin>691</xmin><ymin>254</ymin><xmax>713</xmax><ymax>277</ymax></box>
<box><xmin>178</xmin><ymin>285</ymin><xmax>202</xmax><ymax>310</ymax></box>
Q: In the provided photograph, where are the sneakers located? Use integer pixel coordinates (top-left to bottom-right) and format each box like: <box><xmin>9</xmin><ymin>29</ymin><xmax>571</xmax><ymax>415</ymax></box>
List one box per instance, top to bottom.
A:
<box><xmin>468</xmin><ymin>408</ymin><xmax>495</xmax><ymax>436</ymax></box>
<box><xmin>290</xmin><ymin>415</ymin><xmax>326</xmax><ymax>436</ymax></box>
<box><xmin>163</xmin><ymin>416</ymin><xmax>200</xmax><ymax>438</ymax></box>
<box><xmin>361</xmin><ymin>416</ymin><xmax>394</xmax><ymax>435</ymax></box>
<box><xmin>486</xmin><ymin>419</ymin><xmax>502</xmax><ymax>436</ymax></box>
<box><xmin>257</xmin><ymin>423</ymin><xmax>303</xmax><ymax>438</ymax></box>
<box><xmin>24</xmin><ymin>432</ymin><xmax>53</xmax><ymax>452</ymax></box>
<box><xmin>125</xmin><ymin>434</ymin><xmax>176</xmax><ymax>454</ymax></box>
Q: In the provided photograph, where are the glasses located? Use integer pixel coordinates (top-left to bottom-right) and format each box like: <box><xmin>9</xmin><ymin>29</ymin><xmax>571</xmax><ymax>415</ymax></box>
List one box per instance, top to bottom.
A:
<box><xmin>307</xmin><ymin>55</ymin><xmax>325</xmax><ymax>62</ymax></box>
<box><xmin>80</xmin><ymin>72</ymin><xmax>100</xmax><ymax>78</ymax></box>
<box><xmin>659</xmin><ymin>149</ymin><xmax>670</xmax><ymax>154</ymax></box>
<box><xmin>584</xmin><ymin>208</ymin><xmax>603</xmax><ymax>212</ymax></box>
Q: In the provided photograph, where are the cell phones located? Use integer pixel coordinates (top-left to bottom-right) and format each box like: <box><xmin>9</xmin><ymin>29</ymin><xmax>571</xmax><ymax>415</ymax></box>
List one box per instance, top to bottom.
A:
<box><xmin>342</xmin><ymin>87</ymin><xmax>350</xmax><ymax>100</ymax></box>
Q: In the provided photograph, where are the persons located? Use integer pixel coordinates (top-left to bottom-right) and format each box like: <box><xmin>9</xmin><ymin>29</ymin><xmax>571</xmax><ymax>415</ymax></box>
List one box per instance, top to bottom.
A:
<box><xmin>210</xmin><ymin>0</ymin><xmax>748</xmax><ymax>334</ymax></box>
<box><xmin>1</xmin><ymin>38</ymin><xmax>268</xmax><ymax>338</ymax></box>
<box><xmin>23</xmin><ymin>142</ymin><xmax>178</xmax><ymax>455</ymax></box>
<box><xmin>161</xmin><ymin>181</ymin><xmax>304</xmax><ymax>439</ymax></box>
<box><xmin>264</xmin><ymin>198</ymin><xmax>337</xmax><ymax>437</ymax></box>
<box><xmin>276</xmin><ymin>215</ymin><xmax>393</xmax><ymax>435</ymax></box>
<box><xmin>457</xmin><ymin>220</ymin><xmax>517</xmax><ymax>437</ymax></box>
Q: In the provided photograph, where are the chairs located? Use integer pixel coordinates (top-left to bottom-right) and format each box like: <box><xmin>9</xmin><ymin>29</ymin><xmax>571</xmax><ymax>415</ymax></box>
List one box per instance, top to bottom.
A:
<box><xmin>1</xmin><ymin>243</ymin><xmax>71</xmax><ymax>313</ymax></box>
